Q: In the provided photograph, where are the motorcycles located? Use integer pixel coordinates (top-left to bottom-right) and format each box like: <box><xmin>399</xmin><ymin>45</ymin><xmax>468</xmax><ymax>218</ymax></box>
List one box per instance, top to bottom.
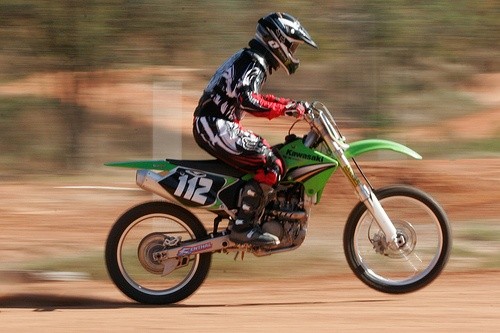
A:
<box><xmin>103</xmin><ymin>101</ymin><xmax>455</xmax><ymax>305</ymax></box>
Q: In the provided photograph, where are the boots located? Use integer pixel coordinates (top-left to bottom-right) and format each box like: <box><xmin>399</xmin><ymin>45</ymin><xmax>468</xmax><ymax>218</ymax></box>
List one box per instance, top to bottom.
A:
<box><xmin>228</xmin><ymin>181</ymin><xmax>281</xmax><ymax>248</ymax></box>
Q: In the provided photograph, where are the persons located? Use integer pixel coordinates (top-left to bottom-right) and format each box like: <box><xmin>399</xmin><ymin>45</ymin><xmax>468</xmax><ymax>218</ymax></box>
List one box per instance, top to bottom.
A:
<box><xmin>192</xmin><ymin>12</ymin><xmax>318</xmax><ymax>246</ymax></box>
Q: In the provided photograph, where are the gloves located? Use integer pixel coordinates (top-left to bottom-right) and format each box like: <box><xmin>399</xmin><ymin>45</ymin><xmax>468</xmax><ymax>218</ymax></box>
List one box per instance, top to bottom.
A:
<box><xmin>285</xmin><ymin>98</ymin><xmax>311</xmax><ymax>117</ymax></box>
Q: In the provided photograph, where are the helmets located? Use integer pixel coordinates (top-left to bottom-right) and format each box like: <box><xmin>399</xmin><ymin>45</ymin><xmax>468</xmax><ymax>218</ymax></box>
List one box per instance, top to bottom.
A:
<box><xmin>254</xmin><ymin>10</ymin><xmax>320</xmax><ymax>77</ymax></box>
